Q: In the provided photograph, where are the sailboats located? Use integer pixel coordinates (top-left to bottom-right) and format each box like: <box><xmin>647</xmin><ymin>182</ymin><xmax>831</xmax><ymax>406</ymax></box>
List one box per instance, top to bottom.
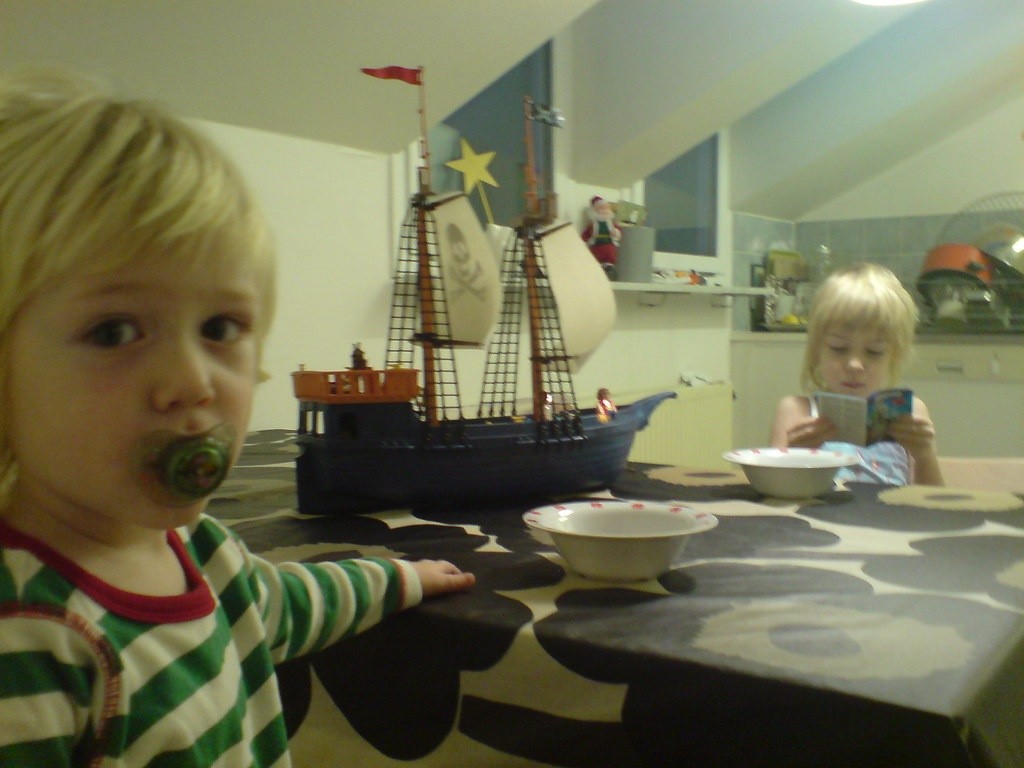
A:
<box><xmin>293</xmin><ymin>64</ymin><xmax>678</xmax><ymax>520</ymax></box>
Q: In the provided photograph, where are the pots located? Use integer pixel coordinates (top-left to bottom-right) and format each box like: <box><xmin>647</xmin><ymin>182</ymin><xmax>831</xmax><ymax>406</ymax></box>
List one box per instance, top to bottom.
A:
<box><xmin>917</xmin><ymin>241</ymin><xmax>994</xmax><ymax>294</ymax></box>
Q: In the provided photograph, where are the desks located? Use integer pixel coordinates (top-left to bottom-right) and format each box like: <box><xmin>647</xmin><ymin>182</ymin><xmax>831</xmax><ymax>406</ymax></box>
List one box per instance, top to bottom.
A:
<box><xmin>199</xmin><ymin>427</ymin><xmax>1024</xmax><ymax>768</ymax></box>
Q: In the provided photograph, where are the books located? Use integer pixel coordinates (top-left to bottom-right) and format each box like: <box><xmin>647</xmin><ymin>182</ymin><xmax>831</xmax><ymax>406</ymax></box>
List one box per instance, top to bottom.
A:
<box><xmin>812</xmin><ymin>389</ymin><xmax>913</xmax><ymax>449</ymax></box>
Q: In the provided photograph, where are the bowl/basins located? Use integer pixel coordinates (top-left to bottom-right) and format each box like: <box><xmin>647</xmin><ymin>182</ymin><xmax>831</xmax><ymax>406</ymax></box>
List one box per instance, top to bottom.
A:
<box><xmin>968</xmin><ymin>223</ymin><xmax>1024</xmax><ymax>274</ymax></box>
<box><xmin>722</xmin><ymin>447</ymin><xmax>859</xmax><ymax>498</ymax></box>
<box><xmin>522</xmin><ymin>501</ymin><xmax>719</xmax><ymax>581</ymax></box>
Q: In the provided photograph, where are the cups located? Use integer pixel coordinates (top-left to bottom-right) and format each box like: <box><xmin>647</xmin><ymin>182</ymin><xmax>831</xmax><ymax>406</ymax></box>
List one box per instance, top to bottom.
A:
<box><xmin>612</xmin><ymin>224</ymin><xmax>655</xmax><ymax>283</ymax></box>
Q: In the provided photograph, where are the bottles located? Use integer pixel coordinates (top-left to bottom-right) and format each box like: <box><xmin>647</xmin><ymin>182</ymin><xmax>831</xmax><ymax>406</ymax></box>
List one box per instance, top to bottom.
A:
<box><xmin>817</xmin><ymin>244</ymin><xmax>832</xmax><ymax>278</ymax></box>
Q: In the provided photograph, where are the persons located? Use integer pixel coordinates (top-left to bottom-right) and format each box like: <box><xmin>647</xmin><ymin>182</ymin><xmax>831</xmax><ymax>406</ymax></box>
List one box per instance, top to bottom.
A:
<box><xmin>1</xmin><ymin>87</ymin><xmax>475</xmax><ymax>768</ymax></box>
<box><xmin>580</xmin><ymin>196</ymin><xmax>623</xmax><ymax>270</ymax></box>
<box><xmin>766</xmin><ymin>262</ymin><xmax>944</xmax><ymax>487</ymax></box>
<box><xmin>597</xmin><ymin>387</ymin><xmax>618</xmax><ymax>422</ymax></box>
